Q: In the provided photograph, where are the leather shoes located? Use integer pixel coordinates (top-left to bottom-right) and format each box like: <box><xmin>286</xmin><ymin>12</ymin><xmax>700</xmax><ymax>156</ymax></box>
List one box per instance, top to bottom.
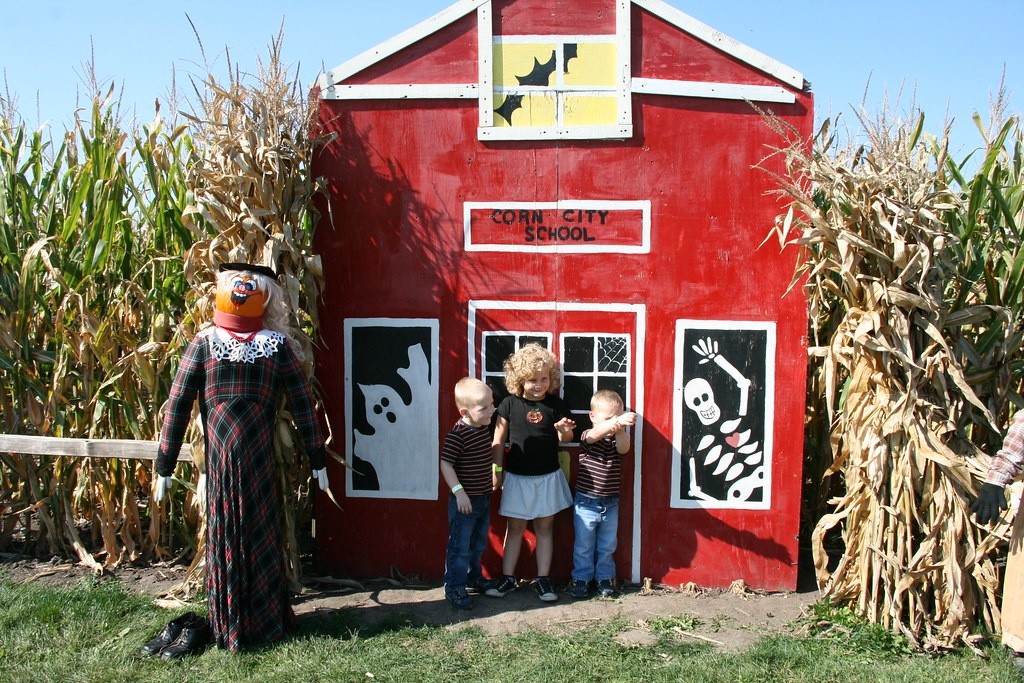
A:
<box><xmin>140</xmin><ymin>611</ymin><xmax>196</xmax><ymax>656</ymax></box>
<box><xmin>160</xmin><ymin>615</ymin><xmax>207</xmax><ymax>661</ymax></box>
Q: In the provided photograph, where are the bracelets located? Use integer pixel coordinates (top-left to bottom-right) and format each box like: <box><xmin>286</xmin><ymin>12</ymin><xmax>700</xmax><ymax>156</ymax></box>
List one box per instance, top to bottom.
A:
<box><xmin>492</xmin><ymin>466</ymin><xmax>503</xmax><ymax>472</ymax></box>
<box><xmin>451</xmin><ymin>484</ymin><xmax>463</xmax><ymax>494</ymax></box>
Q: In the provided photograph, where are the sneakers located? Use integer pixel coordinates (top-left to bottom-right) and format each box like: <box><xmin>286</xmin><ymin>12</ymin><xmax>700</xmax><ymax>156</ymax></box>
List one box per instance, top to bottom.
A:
<box><xmin>465</xmin><ymin>573</ymin><xmax>488</xmax><ymax>594</ymax></box>
<box><xmin>485</xmin><ymin>577</ymin><xmax>516</xmax><ymax>597</ymax></box>
<box><xmin>533</xmin><ymin>577</ymin><xmax>558</xmax><ymax>601</ymax></box>
<box><xmin>570</xmin><ymin>579</ymin><xmax>590</xmax><ymax>597</ymax></box>
<box><xmin>595</xmin><ymin>580</ymin><xmax>615</xmax><ymax>596</ymax></box>
<box><xmin>444</xmin><ymin>582</ymin><xmax>473</xmax><ymax>610</ymax></box>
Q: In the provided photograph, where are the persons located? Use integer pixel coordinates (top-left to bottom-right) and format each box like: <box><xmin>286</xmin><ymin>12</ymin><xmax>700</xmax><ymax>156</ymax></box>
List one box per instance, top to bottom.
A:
<box><xmin>151</xmin><ymin>263</ymin><xmax>330</xmax><ymax>658</ymax></box>
<box><xmin>491</xmin><ymin>342</ymin><xmax>577</xmax><ymax>601</ymax></box>
<box><xmin>441</xmin><ymin>377</ymin><xmax>496</xmax><ymax>611</ymax></box>
<box><xmin>568</xmin><ymin>390</ymin><xmax>638</xmax><ymax>599</ymax></box>
